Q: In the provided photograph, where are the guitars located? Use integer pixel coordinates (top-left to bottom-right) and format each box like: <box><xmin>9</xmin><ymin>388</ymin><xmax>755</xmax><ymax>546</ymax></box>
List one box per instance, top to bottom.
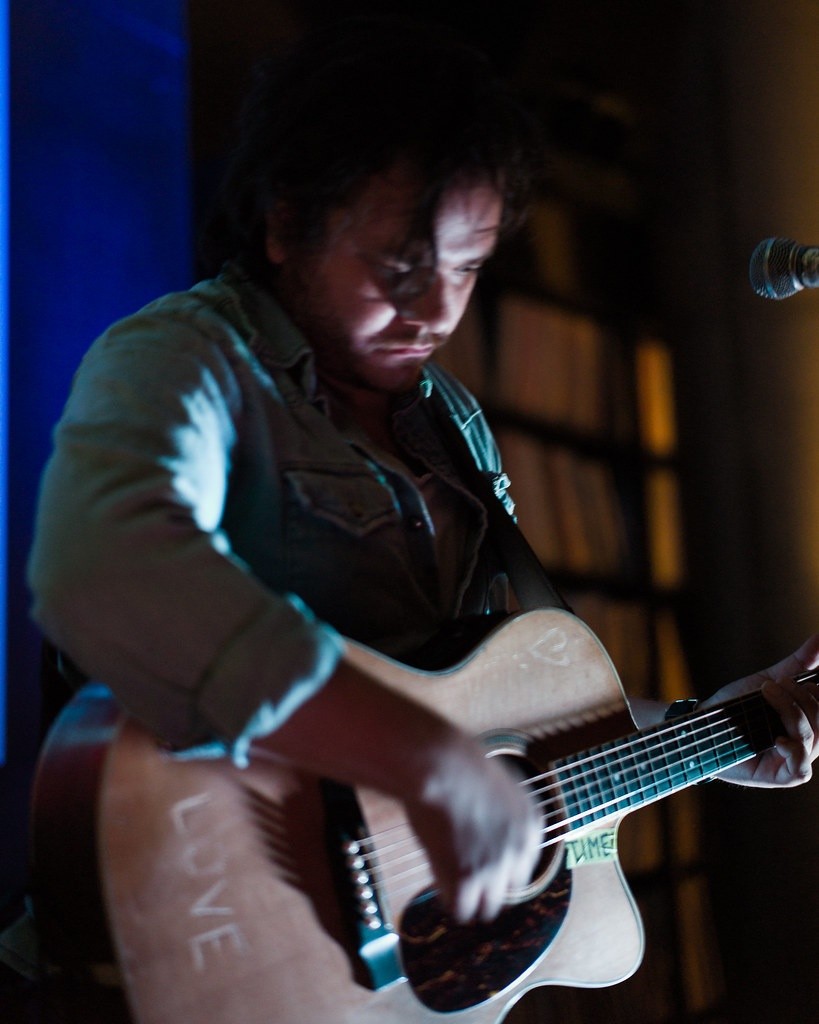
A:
<box><xmin>28</xmin><ymin>606</ymin><xmax>819</xmax><ymax>1024</ymax></box>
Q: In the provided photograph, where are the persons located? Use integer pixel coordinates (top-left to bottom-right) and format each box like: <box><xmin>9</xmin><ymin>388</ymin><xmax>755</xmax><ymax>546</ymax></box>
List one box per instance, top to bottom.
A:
<box><xmin>0</xmin><ymin>29</ymin><xmax>817</xmax><ymax>986</ymax></box>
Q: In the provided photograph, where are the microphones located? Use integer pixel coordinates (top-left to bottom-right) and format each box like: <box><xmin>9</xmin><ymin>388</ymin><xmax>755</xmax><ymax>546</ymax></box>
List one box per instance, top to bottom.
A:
<box><xmin>750</xmin><ymin>236</ymin><xmax>819</xmax><ymax>299</ymax></box>
